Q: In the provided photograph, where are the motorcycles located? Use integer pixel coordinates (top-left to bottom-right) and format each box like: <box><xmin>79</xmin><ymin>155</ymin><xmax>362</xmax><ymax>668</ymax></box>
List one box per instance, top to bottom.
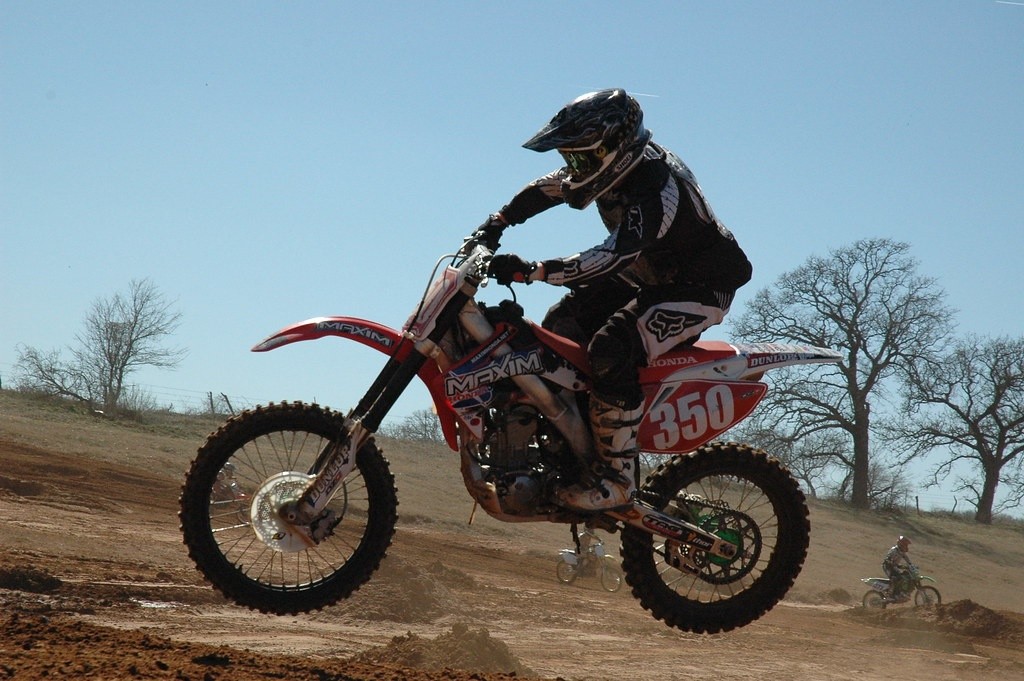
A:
<box><xmin>177</xmin><ymin>224</ymin><xmax>849</xmax><ymax>639</ymax></box>
<box><xmin>555</xmin><ymin>539</ymin><xmax>621</xmax><ymax>595</ymax></box>
<box><xmin>859</xmin><ymin>560</ymin><xmax>942</xmax><ymax>610</ymax></box>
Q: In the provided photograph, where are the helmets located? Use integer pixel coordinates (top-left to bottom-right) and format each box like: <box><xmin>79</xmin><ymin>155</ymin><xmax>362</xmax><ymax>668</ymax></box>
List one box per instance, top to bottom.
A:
<box><xmin>522</xmin><ymin>89</ymin><xmax>653</xmax><ymax>210</ymax></box>
<box><xmin>584</xmin><ymin>525</ymin><xmax>595</xmax><ymax>538</ymax></box>
<box><xmin>896</xmin><ymin>535</ymin><xmax>912</xmax><ymax>552</ymax></box>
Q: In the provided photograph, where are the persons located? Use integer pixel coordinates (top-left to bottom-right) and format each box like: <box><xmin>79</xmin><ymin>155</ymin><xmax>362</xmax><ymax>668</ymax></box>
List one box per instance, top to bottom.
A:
<box><xmin>464</xmin><ymin>87</ymin><xmax>753</xmax><ymax>509</ymax></box>
<box><xmin>575</xmin><ymin>526</ymin><xmax>601</xmax><ymax>577</ymax></box>
<box><xmin>884</xmin><ymin>535</ymin><xmax>914</xmax><ymax>599</ymax></box>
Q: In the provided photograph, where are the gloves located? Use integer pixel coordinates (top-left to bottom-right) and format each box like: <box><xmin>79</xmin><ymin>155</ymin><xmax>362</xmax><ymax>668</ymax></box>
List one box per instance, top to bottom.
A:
<box><xmin>479</xmin><ymin>213</ymin><xmax>505</xmax><ymax>252</ymax></box>
<box><xmin>487</xmin><ymin>254</ymin><xmax>538</xmax><ymax>286</ymax></box>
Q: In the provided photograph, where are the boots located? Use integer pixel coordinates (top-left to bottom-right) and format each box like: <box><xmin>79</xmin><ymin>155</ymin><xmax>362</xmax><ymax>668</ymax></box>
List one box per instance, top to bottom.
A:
<box><xmin>551</xmin><ymin>394</ymin><xmax>647</xmax><ymax>515</ymax></box>
